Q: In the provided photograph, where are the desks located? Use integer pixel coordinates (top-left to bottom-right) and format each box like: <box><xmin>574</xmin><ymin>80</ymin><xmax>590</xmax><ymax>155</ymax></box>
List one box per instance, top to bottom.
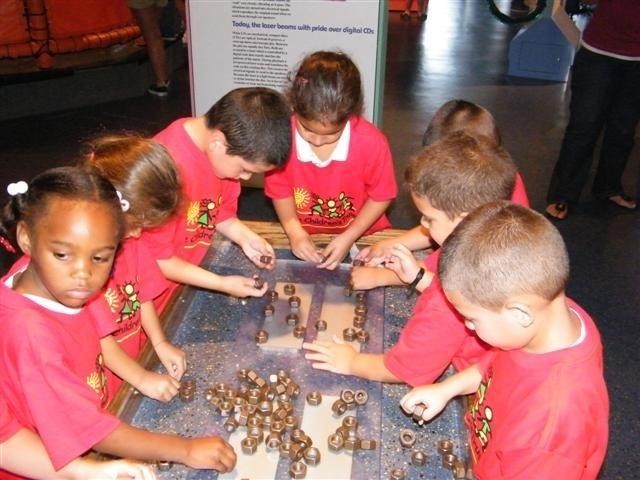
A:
<box><xmin>85</xmin><ymin>220</ymin><xmax>472</xmax><ymax>480</ymax></box>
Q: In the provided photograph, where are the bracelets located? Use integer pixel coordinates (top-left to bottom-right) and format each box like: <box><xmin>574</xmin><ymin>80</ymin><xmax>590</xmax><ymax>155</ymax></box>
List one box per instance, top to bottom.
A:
<box><xmin>407</xmin><ymin>267</ymin><xmax>425</xmax><ymax>288</ymax></box>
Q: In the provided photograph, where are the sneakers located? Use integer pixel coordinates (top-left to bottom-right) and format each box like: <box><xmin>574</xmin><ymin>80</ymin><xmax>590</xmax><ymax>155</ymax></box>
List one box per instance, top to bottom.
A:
<box><xmin>401</xmin><ymin>12</ymin><xmax>428</xmax><ymax>21</ymax></box>
<box><xmin>148</xmin><ymin>80</ymin><xmax>172</xmax><ymax>97</ymax></box>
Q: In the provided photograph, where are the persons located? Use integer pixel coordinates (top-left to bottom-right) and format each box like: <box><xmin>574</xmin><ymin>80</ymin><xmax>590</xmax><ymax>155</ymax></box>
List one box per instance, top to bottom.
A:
<box><xmin>126</xmin><ymin>0</ymin><xmax>170</xmax><ymax>98</ymax></box>
<box><xmin>400</xmin><ymin>0</ymin><xmax>427</xmax><ymax>22</ymax></box>
<box><xmin>544</xmin><ymin>0</ymin><xmax>639</xmax><ymax>220</ymax></box>
<box><xmin>263</xmin><ymin>48</ymin><xmax>399</xmax><ymax>273</ymax></box>
<box><xmin>133</xmin><ymin>85</ymin><xmax>294</xmax><ymax>343</ymax></box>
<box><xmin>348</xmin><ymin>97</ymin><xmax>532</xmax><ymax>294</ymax></box>
<box><xmin>50</xmin><ymin>128</ymin><xmax>190</xmax><ymax>405</ymax></box>
<box><xmin>301</xmin><ymin>128</ymin><xmax>520</xmax><ymax>392</ymax></box>
<box><xmin>1</xmin><ymin>163</ymin><xmax>239</xmax><ymax>474</ymax></box>
<box><xmin>396</xmin><ymin>198</ymin><xmax>611</xmax><ymax>480</ymax></box>
<box><xmin>1</xmin><ymin>393</ymin><xmax>161</xmax><ymax>480</ymax></box>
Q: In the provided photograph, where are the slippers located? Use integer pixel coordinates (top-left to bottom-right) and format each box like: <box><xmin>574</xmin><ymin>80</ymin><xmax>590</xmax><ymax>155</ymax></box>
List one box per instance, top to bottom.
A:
<box><xmin>600</xmin><ymin>194</ymin><xmax>637</xmax><ymax>212</ymax></box>
<box><xmin>547</xmin><ymin>202</ymin><xmax>568</xmax><ymax>221</ymax></box>
